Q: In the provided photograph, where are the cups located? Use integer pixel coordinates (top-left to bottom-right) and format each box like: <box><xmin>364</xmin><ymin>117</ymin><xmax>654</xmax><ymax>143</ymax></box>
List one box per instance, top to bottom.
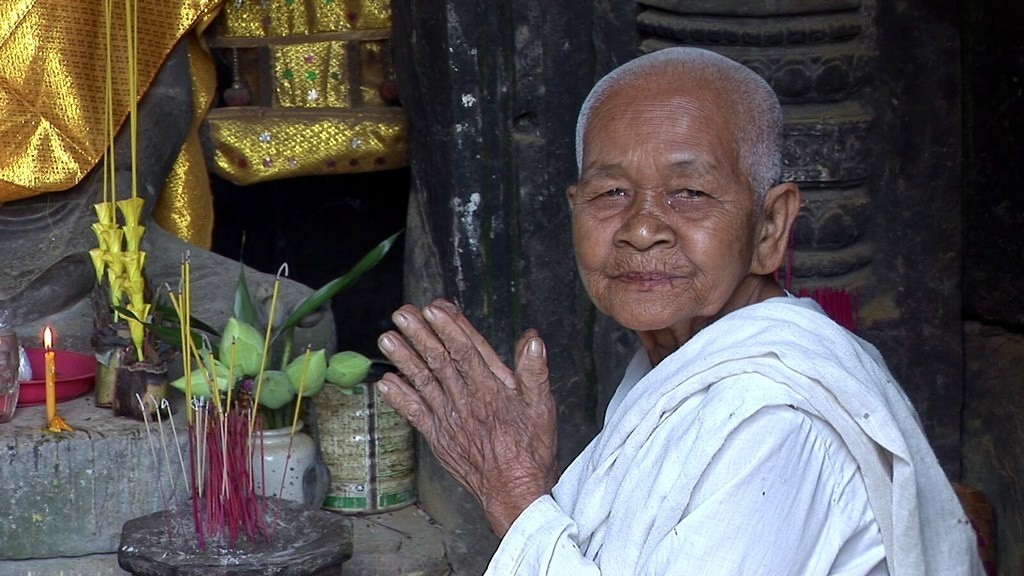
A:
<box><xmin>0</xmin><ymin>329</ymin><xmax>21</xmax><ymax>423</ymax></box>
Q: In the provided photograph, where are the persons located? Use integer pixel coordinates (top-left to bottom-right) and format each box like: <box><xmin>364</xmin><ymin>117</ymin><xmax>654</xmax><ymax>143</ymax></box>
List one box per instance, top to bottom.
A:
<box><xmin>376</xmin><ymin>48</ymin><xmax>989</xmax><ymax>576</ymax></box>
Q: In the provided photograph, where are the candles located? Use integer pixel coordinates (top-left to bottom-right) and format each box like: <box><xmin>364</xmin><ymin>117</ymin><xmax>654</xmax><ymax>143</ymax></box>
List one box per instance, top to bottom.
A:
<box><xmin>43</xmin><ymin>328</ymin><xmax>58</xmax><ymax>432</ymax></box>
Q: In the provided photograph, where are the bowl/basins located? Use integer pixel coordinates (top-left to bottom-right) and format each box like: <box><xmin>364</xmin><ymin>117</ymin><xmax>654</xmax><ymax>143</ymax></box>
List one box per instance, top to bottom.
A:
<box><xmin>16</xmin><ymin>346</ymin><xmax>98</xmax><ymax>408</ymax></box>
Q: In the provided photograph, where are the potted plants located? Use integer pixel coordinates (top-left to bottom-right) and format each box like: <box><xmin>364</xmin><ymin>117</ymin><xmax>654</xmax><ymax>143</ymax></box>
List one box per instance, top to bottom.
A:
<box><xmin>102</xmin><ymin>236</ymin><xmax>403</xmax><ymax>507</ymax></box>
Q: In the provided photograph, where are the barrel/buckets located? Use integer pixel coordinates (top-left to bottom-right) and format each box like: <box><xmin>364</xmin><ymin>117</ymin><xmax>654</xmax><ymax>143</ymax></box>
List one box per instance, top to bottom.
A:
<box><xmin>305</xmin><ymin>360</ymin><xmax>417</xmax><ymax>514</ymax></box>
